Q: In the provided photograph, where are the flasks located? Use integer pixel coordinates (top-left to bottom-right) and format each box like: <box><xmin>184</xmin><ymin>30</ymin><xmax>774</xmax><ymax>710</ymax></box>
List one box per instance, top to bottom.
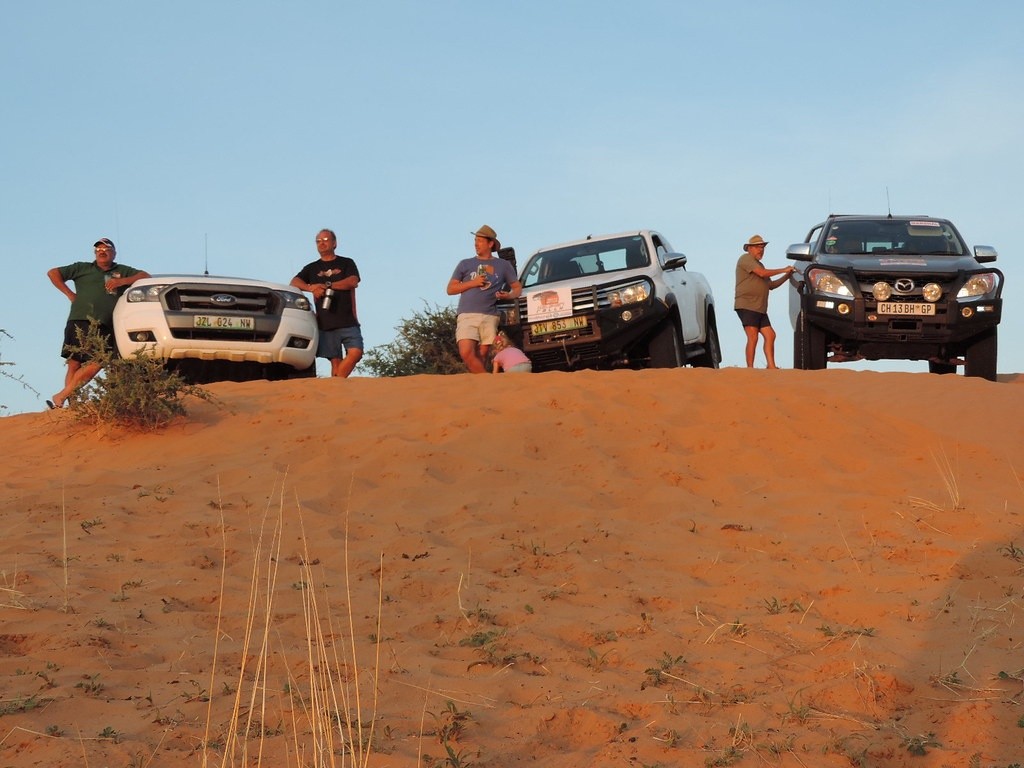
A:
<box><xmin>322</xmin><ymin>289</ymin><xmax>335</xmax><ymax>310</ymax></box>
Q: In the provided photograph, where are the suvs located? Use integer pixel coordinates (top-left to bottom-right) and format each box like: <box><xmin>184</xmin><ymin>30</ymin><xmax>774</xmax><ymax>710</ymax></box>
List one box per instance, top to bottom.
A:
<box><xmin>786</xmin><ymin>214</ymin><xmax>1006</xmax><ymax>381</ymax></box>
<box><xmin>496</xmin><ymin>227</ymin><xmax>724</xmax><ymax>372</ymax></box>
<box><xmin>111</xmin><ymin>271</ymin><xmax>320</xmax><ymax>380</ymax></box>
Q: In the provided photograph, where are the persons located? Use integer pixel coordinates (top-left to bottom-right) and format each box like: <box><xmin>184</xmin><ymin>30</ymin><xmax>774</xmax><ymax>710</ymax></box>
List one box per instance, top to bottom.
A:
<box><xmin>447</xmin><ymin>225</ymin><xmax>522</xmax><ymax>373</ymax></box>
<box><xmin>289</xmin><ymin>229</ymin><xmax>364</xmax><ymax>377</ymax></box>
<box><xmin>734</xmin><ymin>236</ymin><xmax>794</xmax><ymax>369</ymax></box>
<box><xmin>46</xmin><ymin>238</ymin><xmax>151</xmax><ymax>409</ymax></box>
<box><xmin>491</xmin><ymin>331</ymin><xmax>532</xmax><ymax>373</ymax></box>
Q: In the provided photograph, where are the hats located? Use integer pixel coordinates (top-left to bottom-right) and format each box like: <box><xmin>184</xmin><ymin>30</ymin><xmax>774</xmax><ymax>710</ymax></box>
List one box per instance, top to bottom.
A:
<box><xmin>471</xmin><ymin>225</ymin><xmax>500</xmax><ymax>251</ymax></box>
<box><xmin>744</xmin><ymin>235</ymin><xmax>769</xmax><ymax>251</ymax></box>
<box><xmin>94</xmin><ymin>238</ymin><xmax>114</xmax><ymax>250</ymax></box>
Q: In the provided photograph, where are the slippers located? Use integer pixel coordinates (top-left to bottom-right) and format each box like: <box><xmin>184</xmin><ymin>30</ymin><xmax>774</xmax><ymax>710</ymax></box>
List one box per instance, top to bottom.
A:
<box><xmin>46</xmin><ymin>400</ymin><xmax>55</xmax><ymax>409</ymax></box>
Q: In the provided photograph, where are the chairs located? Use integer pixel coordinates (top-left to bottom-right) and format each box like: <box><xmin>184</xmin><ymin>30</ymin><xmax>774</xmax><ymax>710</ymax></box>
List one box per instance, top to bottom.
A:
<box><xmin>836</xmin><ymin>236</ymin><xmax>862</xmax><ymax>253</ymax></box>
<box><xmin>907</xmin><ymin>236</ymin><xmax>951</xmax><ymax>252</ymax></box>
<box><xmin>625</xmin><ymin>250</ymin><xmax>646</xmax><ymax>267</ymax></box>
<box><xmin>551</xmin><ymin>261</ymin><xmax>581</xmax><ymax>278</ymax></box>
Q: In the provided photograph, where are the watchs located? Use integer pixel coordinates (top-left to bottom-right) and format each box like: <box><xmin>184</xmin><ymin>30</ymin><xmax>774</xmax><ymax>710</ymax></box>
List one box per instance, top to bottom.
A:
<box><xmin>325</xmin><ymin>281</ymin><xmax>332</xmax><ymax>289</ymax></box>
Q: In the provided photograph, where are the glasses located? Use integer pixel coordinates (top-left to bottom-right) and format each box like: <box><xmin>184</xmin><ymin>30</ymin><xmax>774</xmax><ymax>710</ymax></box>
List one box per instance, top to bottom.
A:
<box><xmin>317</xmin><ymin>237</ymin><xmax>334</xmax><ymax>242</ymax></box>
<box><xmin>96</xmin><ymin>247</ymin><xmax>112</xmax><ymax>253</ymax></box>
